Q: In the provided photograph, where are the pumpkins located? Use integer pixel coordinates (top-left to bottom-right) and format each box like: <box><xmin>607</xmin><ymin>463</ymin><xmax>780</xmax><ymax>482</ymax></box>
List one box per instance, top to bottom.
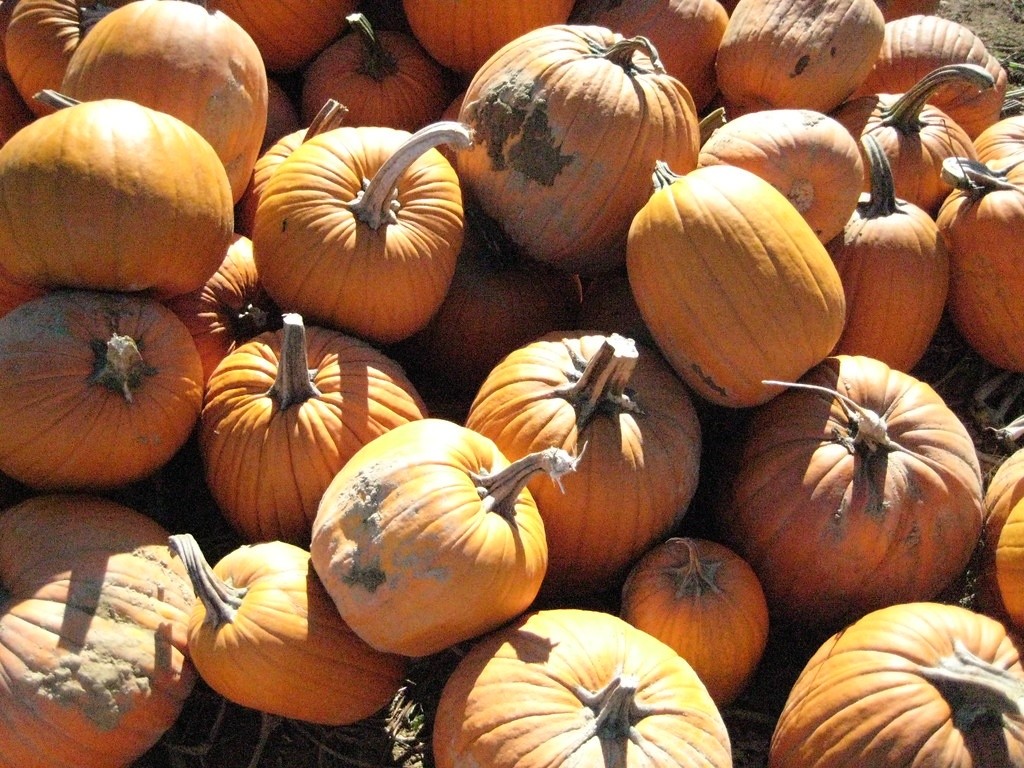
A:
<box><xmin>0</xmin><ymin>1</ymin><xmax>1024</xmax><ymax>768</ymax></box>
